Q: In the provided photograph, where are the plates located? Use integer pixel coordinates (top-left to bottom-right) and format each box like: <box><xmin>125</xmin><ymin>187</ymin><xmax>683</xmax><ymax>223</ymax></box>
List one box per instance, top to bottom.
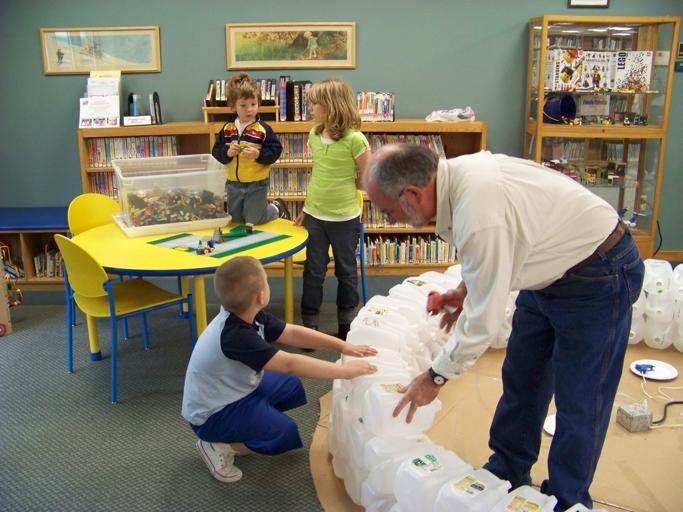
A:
<box><xmin>630</xmin><ymin>359</ymin><xmax>678</xmax><ymax>380</ymax></box>
<box><xmin>542</xmin><ymin>414</ymin><xmax>556</xmax><ymax>436</ymax></box>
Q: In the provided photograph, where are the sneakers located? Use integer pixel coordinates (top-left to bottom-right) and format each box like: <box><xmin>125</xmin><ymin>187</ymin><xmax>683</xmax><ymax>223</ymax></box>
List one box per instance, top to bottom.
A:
<box><xmin>274</xmin><ymin>197</ymin><xmax>293</xmax><ymax>220</ymax></box>
<box><xmin>338</xmin><ymin>326</ymin><xmax>348</xmax><ymax>339</ymax></box>
<box><xmin>301</xmin><ymin>328</ymin><xmax>319</xmax><ymax>352</ymax></box>
<box><xmin>199</xmin><ymin>439</ymin><xmax>242</xmax><ymax>482</ymax></box>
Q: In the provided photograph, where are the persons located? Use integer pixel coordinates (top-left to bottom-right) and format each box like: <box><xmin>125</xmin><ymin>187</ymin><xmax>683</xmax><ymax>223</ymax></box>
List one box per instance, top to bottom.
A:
<box><xmin>303</xmin><ymin>31</ymin><xmax>318</xmax><ymax>60</ymax></box>
<box><xmin>56</xmin><ymin>49</ymin><xmax>64</xmax><ymax>63</ymax></box>
<box><xmin>212</xmin><ymin>71</ymin><xmax>291</xmax><ymax>226</ymax></box>
<box><xmin>294</xmin><ymin>79</ymin><xmax>371</xmax><ymax>354</ymax></box>
<box><xmin>360</xmin><ymin>140</ymin><xmax>645</xmax><ymax>511</ymax></box>
<box><xmin>180</xmin><ymin>256</ymin><xmax>379</xmax><ymax>483</ymax></box>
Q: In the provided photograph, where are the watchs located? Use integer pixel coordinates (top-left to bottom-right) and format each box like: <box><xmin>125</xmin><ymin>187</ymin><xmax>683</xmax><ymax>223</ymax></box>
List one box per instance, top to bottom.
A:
<box><xmin>427</xmin><ymin>367</ymin><xmax>447</xmax><ymax>387</ymax></box>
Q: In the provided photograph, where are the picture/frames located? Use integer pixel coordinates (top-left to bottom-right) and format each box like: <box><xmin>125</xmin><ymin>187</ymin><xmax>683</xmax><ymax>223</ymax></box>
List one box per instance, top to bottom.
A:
<box><xmin>225</xmin><ymin>22</ymin><xmax>358</xmax><ymax>72</ymax></box>
<box><xmin>40</xmin><ymin>24</ymin><xmax>160</xmax><ymax>75</ymax></box>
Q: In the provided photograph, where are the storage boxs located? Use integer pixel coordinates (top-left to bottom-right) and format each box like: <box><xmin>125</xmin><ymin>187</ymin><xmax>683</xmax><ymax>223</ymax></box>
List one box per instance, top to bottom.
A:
<box><xmin>111</xmin><ymin>153</ymin><xmax>229</xmax><ymax>237</ymax></box>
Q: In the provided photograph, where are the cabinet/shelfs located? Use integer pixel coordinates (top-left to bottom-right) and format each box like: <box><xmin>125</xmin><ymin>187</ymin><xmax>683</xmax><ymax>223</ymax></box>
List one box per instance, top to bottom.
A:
<box><xmin>523</xmin><ymin>17</ymin><xmax>679</xmax><ymax>258</ymax></box>
<box><xmin>77</xmin><ymin>121</ymin><xmax>488</xmax><ymax>277</ymax></box>
<box><xmin>0</xmin><ymin>207</ymin><xmax>73</xmax><ymax>291</ymax></box>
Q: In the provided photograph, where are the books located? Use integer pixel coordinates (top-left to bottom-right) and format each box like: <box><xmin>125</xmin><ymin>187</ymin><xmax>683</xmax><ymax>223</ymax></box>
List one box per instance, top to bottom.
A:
<box><xmin>78</xmin><ymin>69</ymin><xmax>120</xmax><ymax>128</ymax></box>
<box><xmin>280</xmin><ymin>76</ymin><xmax>312</xmax><ymax>121</ymax></box>
<box><xmin>206</xmin><ymin>78</ymin><xmax>277</xmax><ymax>106</ymax></box>
<box><xmin>356</xmin><ymin>91</ymin><xmax>395</xmax><ymax>122</ymax></box>
<box><xmin>277</xmin><ymin>133</ymin><xmax>315</xmax><ymax>163</ymax></box>
<box><xmin>365</xmin><ymin>134</ymin><xmax>447</xmax><ymax>158</ymax></box>
<box><xmin>88</xmin><ymin>137</ymin><xmax>178</xmax><ymax>168</ymax></box>
<box><xmin>268</xmin><ymin>167</ymin><xmax>313</xmax><ymax>196</ymax></box>
<box><xmin>91</xmin><ymin>171</ymin><xmax>117</xmax><ymax>199</ymax></box>
<box><xmin>286</xmin><ymin>201</ymin><xmax>304</xmax><ymax>221</ymax></box>
<box><xmin>362</xmin><ymin>201</ymin><xmax>413</xmax><ymax>229</ymax></box>
<box><xmin>359</xmin><ymin>236</ymin><xmax>457</xmax><ymax>264</ymax></box>
<box><xmin>33</xmin><ymin>244</ymin><xmax>62</xmax><ymax>279</ymax></box>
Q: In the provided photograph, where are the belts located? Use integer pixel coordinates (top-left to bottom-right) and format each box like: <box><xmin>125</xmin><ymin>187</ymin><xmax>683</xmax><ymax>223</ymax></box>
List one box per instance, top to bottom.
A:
<box><xmin>569</xmin><ymin>223</ymin><xmax>626</xmax><ymax>275</ymax></box>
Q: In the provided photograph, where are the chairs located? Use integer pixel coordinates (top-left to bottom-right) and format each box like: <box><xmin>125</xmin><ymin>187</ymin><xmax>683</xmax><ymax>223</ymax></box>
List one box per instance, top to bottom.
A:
<box><xmin>276</xmin><ymin>189</ymin><xmax>369</xmax><ymax>305</ymax></box>
<box><xmin>66</xmin><ymin>192</ymin><xmax>122</xmax><ymax>326</ymax></box>
<box><xmin>53</xmin><ymin>234</ymin><xmax>195</xmax><ymax>403</ymax></box>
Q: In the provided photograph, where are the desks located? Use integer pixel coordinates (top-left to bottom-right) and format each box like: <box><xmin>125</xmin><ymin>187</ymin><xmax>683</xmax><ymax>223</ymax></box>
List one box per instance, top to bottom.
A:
<box><xmin>67</xmin><ymin>216</ymin><xmax>312</xmax><ymax>359</ymax></box>
